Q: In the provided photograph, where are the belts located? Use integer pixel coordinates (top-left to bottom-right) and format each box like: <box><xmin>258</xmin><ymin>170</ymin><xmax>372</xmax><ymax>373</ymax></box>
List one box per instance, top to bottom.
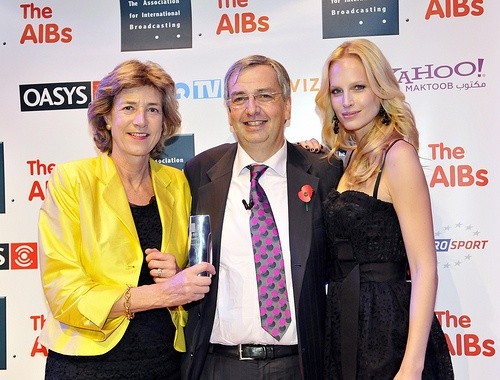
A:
<box><xmin>208</xmin><ymin>343</ymin><xmax>299</xmax><ymax>361</ymax></box>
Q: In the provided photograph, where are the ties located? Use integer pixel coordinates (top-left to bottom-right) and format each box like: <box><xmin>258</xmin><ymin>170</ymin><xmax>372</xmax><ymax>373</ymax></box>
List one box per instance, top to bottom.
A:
<box><xmin>246</xmin><ymin>164</ymin><xmax>292</xmax><ymax>342</ymax></box>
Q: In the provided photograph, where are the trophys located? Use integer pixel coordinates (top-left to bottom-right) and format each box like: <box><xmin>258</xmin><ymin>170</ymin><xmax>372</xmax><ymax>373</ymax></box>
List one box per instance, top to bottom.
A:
<box><xmin>189</xmin><ymin>214</ymin><xmax>211</xmax><ymax>277</ymax></box>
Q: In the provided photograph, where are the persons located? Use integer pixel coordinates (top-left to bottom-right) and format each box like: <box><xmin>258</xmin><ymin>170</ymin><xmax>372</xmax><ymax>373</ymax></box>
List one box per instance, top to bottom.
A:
<box><xmin>294</xmin><ymin>39</ymin><xmax>454</xmax><ymax>380</ymax></box>
<box><xmin>177</xmin><ymin>55</ymin><xmax>344</xmax><ymax>380</ymax></box>
<box><xmin>38</xmin><ymin>58</ymin><xmax>216</xmax><ymax>380</ymax></box>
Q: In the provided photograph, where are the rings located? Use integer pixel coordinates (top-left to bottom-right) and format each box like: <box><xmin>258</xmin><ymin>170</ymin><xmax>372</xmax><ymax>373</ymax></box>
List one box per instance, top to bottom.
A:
<box><xmin>157</xmin><ymin>269</ymin><xmax>162</xmax><ymax>277</ymax></box>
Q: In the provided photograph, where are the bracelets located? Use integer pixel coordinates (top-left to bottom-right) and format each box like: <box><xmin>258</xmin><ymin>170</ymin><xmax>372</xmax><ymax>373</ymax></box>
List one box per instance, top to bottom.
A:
<box><xmin>124</xmin><ymin>286</ymin><xmax>134</xmax><ymax>320</ymax></box>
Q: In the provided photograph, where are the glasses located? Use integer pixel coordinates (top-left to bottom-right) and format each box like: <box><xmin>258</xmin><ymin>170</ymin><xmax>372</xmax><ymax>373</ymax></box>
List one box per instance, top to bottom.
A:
<box><xmin>226</xmin><ymin>92</ymin><xmax>284</xmax><ymax>108</ymax></box>
